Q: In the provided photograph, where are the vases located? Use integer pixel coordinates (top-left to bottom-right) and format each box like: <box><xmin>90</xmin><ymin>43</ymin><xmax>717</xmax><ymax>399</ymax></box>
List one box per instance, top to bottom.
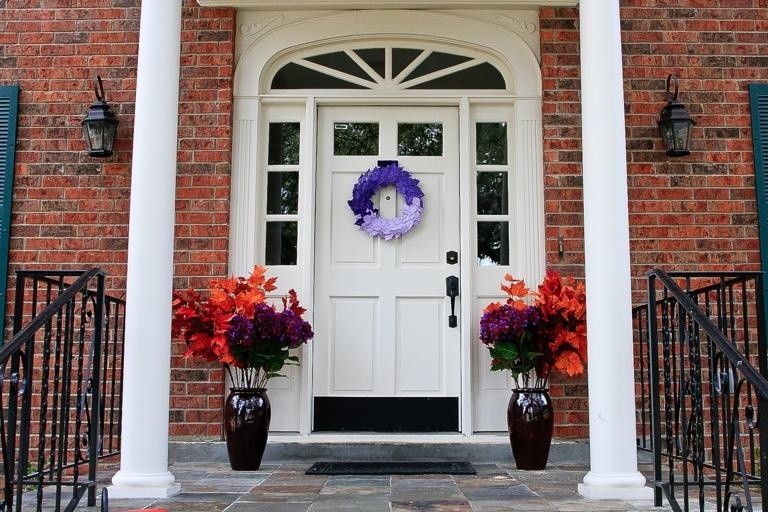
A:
<box><xmin>223</xmin><ymin>387</ymin><xmax>272</xmax><ymax>472</ymax></box>
<box><xmin>505</xmin><ymin>389</ymin><xmax>556</xmax><ymax>471</ymax></box>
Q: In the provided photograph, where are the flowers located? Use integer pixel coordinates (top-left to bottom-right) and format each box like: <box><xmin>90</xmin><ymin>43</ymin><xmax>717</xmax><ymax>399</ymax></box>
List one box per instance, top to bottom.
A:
<box><xmin>346</xmin><ymin>163</ymin><xmax>426</xmax><ymax>242</ymax></box>
<box><xmin>171</xmin><ymin>263</ymin><xmax>315</xmax><ymax>387</ymax></box>
<box><xmin>478</xmin><ymin>267</ymin><xmax>590</xmax><ymax>388</ymax></box>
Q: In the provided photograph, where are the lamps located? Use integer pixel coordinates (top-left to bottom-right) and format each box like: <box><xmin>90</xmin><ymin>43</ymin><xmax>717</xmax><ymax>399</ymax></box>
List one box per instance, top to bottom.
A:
<box><xmin>79</xmin><ymin>73</ymin><xmax>120</xmax><ymax>159</ymax></box>
<box><xmin>655</xmin><ymin>73</ymin><xmax>699</xmax><ymax>159</ymax></box>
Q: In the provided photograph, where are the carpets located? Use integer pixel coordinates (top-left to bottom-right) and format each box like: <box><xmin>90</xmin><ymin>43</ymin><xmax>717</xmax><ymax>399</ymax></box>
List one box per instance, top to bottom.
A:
<box><xmin>304</xmin><ymin>460</ymin><xmax>478</xmax><ymax>477</ymax></box>
